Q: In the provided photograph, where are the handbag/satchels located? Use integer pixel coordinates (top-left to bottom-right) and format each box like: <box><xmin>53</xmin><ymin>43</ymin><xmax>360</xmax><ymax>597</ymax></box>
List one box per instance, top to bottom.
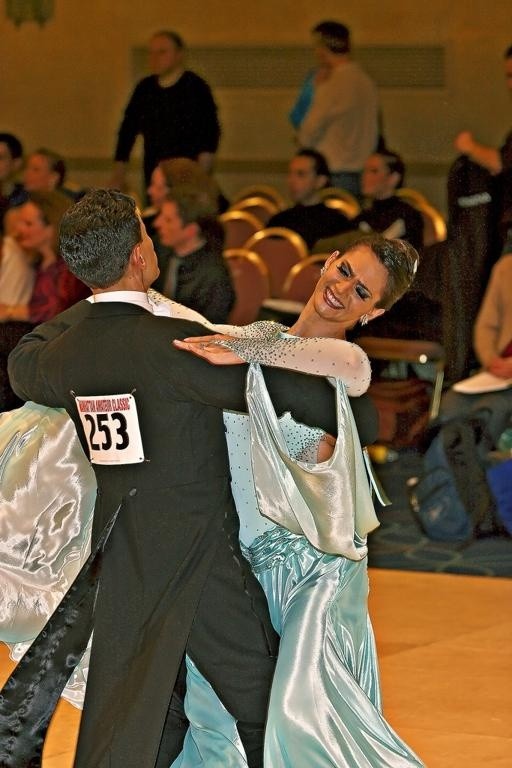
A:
<box><xmin>409</xmin><ymin>405</ymin><xmax>493</xmax><ymax>542</ymax></box>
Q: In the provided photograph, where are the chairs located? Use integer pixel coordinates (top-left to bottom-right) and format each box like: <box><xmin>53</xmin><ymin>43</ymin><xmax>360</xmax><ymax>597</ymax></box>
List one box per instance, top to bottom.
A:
<box><xmin>61</xmin><ymin>181</ymin><xmax>448</xmax><ymax>425</ymax></box>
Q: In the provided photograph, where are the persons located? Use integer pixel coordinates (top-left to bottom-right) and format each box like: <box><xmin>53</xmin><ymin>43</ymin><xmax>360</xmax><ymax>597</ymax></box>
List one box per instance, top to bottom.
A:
<box><xmin>300</xmin><ymin>20</ymin><xmax>382</xmax><ymax>202</ymax></box>
<box><xmin>289</xmin><ymin>22</ymin><xmax>385</xmax><ymax>154</ymax></box>
<box><xmin>113</xmin><ymin>29</ymin><xmax>221</xmax><ymax>210</ymax></box>
<box><xmin>454</xmin><ymin>43</ymin><xmax>512</xmax><ymax>176</ymax></box>
<box><xmin>0</xmin><ymin>132</ymin><xmax>511</xmax><ymax>539</ymax></box>
<box><xmin>0</xmin><ymin>188</ymin><xmax>346</xmax><ymax>767</ymax></box>
<box><xmin>0</xmin><ymin>234</ymin><xmax>427</xmax><ymax>767</ymax></box>
